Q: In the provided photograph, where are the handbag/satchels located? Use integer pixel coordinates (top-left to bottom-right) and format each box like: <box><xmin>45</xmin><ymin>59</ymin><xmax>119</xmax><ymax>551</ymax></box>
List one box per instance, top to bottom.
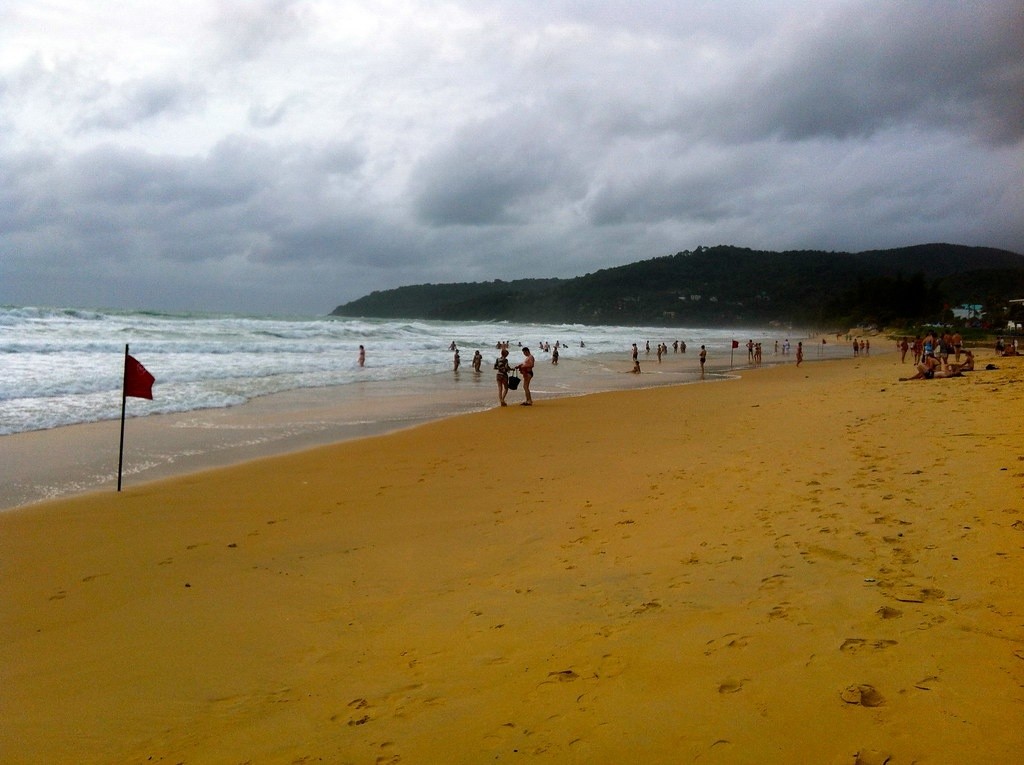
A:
<box><xmin>509</xmin><ymin>367</ymin><xmax>521</xmax><ymax>390</ymax></box>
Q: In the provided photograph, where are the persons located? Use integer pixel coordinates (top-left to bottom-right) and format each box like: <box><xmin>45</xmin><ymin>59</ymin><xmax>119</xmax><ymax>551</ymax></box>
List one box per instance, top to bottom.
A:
<box><xmin>897</xmin><ymin>329</ymin><xmax>974</xmax><ymax>381</ymax></box>
<box><xmin>837</xmin><ymin>333</ymin><xmax>870</xmax><ymax>357</ymax></box>
<box><xmin>994</xmin><ymin>336</ymin><xmax>1020</xmax><ymax>356</ymax></box>
<box><xmin>496</xmin><ymin>339</ymin><xmax>586</xmax><ymax>364</ymax></box>
<box><xmin>626</xmin><ymin>340</ymin><xmax>686</xmax><ymax>373</ymax></box>
<box><xmin>746</xmin><ymin>340</ymin><xmax>790</xmax><ymax>364</ymax></box>
<box><xmin>449</xmin><ymin>341</ymin><xmax>457</xmax><ymax>351</ymax></box>
<box><xmin>796</xmin><ymin>342</ymin><xmax>802</xmax><ymax>367</ymax></box>
<box><xmin>357</xmin><ymin>345</ymin><xmax>365</xmax><ymax>367</ymax></box>
<box><xmin>699</xmin><ymin>345</ymin><xmax>707</xmax><ymax>376</ymax></box>
<box><xmin>515</xmin><ymin>347</ymin><xmax>535</xmax><ymax>406</ymax></box>
<box><xmin>453</xmin><ymin>349</ymin><xmax>461</xmax><ymax>371</ymax></box>
<box><xmin>494</xmin><ymin>349</ymin><xmax>515</xmax><ymax>407</ymax></box>
<box><xmin>472</xmin><ymin>350</ymin><xmax>482</xmax><ymax>372</ymax></box>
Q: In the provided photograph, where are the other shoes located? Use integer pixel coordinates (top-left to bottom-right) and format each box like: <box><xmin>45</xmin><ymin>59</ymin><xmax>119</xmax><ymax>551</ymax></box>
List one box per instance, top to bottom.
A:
<box><xmin>501</xmin><ymin>402</ymin><xmax>507</xmax><ymax>406</ymax></box>
<box><xmin>521</xmin><ymin>402</ymin><xmax>532</xmax><ymax>405</ymax></box>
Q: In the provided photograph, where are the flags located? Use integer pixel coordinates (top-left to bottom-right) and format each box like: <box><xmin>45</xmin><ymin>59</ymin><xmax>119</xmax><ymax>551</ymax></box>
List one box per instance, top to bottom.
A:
<box><xmin>732</xmin><ymin>340</ymin><xmax>739</xmax><ymax>348</ymax></box>
<box><xmin>125</xmin><ymin>355</ymin><xmax>156</xmax><ymax>400</ymax></box>
